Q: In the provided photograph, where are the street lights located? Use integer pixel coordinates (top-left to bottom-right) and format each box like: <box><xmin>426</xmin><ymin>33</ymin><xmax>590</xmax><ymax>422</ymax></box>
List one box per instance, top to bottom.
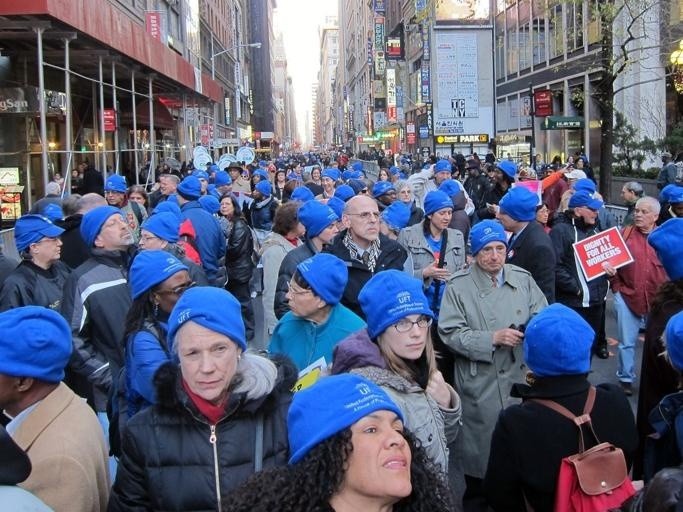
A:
<box><xmin>210</xmin><ymin>42</ymin><xmax>265</xmax><ymax>79</ymax></box>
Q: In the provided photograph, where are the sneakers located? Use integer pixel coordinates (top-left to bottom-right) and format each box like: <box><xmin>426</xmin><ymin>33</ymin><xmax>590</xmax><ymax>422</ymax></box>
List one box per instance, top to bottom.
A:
<box><xmin>595</xmin><ymin>347</ymin><xmax>610</xmax><ymax>359</ymax></box>
<box><xmin>619</xmin><ymin>378</ymin><xmax>635</xmax><ymax>396</ymax></box>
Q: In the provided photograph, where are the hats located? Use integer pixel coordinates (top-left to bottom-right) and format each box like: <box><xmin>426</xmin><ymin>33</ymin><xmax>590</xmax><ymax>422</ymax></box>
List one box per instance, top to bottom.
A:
<box><xmin>433</xmin><ymin>159</ymin><xmax>451</xmax><ymax>174</ymax></box>
<box><xmin>464</xmin><ymin>159</ymin><xmax>479</xmax><ymax>170</ymax></box>
<box><xmin>350</xmin><ymin>160</ymin><xmax>364</xmax><ymax>172</ymax></box>
<box><xmin>495</xmin><ymin>160</ymin><xmax>517</xmax><ymax>180</ymax></box>
<box><xmin>225</xmin><ymin>162</ymin><xmax>243</xmax><ymax>174</ymax></box>
<box><xmin>210</xmin><ymin>165</ymin><xmax>220</xmax><ymax>173</ymax></box>
<box><xmin>389</xmin><ymin>166</ymin><xmax>398</xmax><ymax>175</ymax></box>
<box><xmin>320</xmin><ymin>167</ymin><xmax>339</xmax><ymax>180</ymax></box>
<box><xmin>192</xmin><ymin>169</ymin><xmax>210</xmax><ymax>184</ymax></box>
<box><xmin>252</xmin><ymin>169</ymin><xmax>268</xmax><ymax>180</ymax></box>
<box><xmin>342</xmin><ymin>170</ymin><xmax>350</xmax><ymax>180</ymax></box>
<box><xmin>350</xmin><ymin>170</ymin><xmax>364</xmax><ymax>178</ymax></box>
<box><xmin>214</xmin><ymin>171</ymin><xmax>232</xmax><ymax>187</ymax></box>
<box><xmin>399</xmin><ymin>172</ymin><xmax>407</xmax><ymax>180</ymax></box>
<box><xmin>103</xmin><ymin>175</ymin><xmax>127</xmax><ymax>193</ymax></box>
<box><xmin>177</xmin><ymin>175</ymin><xmax>201</xmax><ymax>199</ymax></box>
<box><xmin>347</xmin><ymin>178</ymin><xmax>367</xmax><ymax>195</ymax></box>
<box><xmin>572</xmin><ymin>178</ymin><xmax>596</xmax><ymax>194</ymax></box>
<box><xmin>255</xmin><ymin>180</ymin><xmax>273</xmax><ymax>197</ymax></box>
<box><xmin>370</xmin><ymin>180</ymin><xmax>396</xmax><ymax>198</ymax></box>
<box><xmin>437</xmin><ymin>180</ymin><xmax>461</xmax><ymax>199</ymax></box>
<box><xmin>658</xmin><ymin>183</ymin><xmax>676</xmax><ymax>204</ymax></box>
<box><xmin>205</xmin><ymin>184</ymin><xmax>216</xmax><ymax>194</ymax></box>
<box><xmin>334</xmin><ymin>184</ymin><xmax>355</xmax><ymax>201</ymax></box>
<box><xmin>290</xmin><ymin>186</ymin><xmax>314</xmax><ymax>203</ymax></box>
<box><xmin>498</xmin><ymin>186</ymin><xmax>539</xmax><ymax>222</ymax></box>
<box><xmin>667</xmin><ymin>186</ymin><xmax>683</xmax><ymax>203</ymax></box>
<box><xmin>424</xmin><ymin>190</ymin><xmax>453</xmax><ymax>216</ymax></box>
<box><xmin>568</xmin><ymin>190</ymin><xmax>603</xmax><ymax>210</ymax></box>
<box><xmin>166</xmin><ymin>194</ymin><xmax>179</xmax><ymax>207</ymax></box>
<box><xmin>198</xmin><ymin>195</ymin><xmax>220</xmax><ymax>216</ymax></box>
<box><xmin>326</xmin><ymin>197</ymin><xmax>345</xmax><ymax>220</ymax></box>
<box><xmin>297</xmin><ymin>199</ymin><xmax>339</xmax><ymax>239</ymax></box>
<box><xmin>380</xmin><ymin>201</ymin><xmax>412</xmax><ymax>234</ymax></box>
<box><xmin>150</xmin><ymin>202</ymin><xmax>182</xmax><ymax>225</ymax></box>
<box><xmin>78</xmin><ymin>206</ymin><xmax>126</xmax><ymax>249</ymax></box>
<box><xmin>140</xmin><ymin>211</ymin><xmax>179</xmax><ymax>244</ymax></box>
<box><xmin>14</xmin><ymin>214</ymin><xmax>66</xmax><ymax>254</ymax></box>
<box><xmin>646</xmin><ymin>217</ymin><xmax>682</xmax><ymax>281</ymax></box>
<box><xmin>469</xmin><ymin>219</ymin><xmax>508</xmax><ymax>257</ymax></box>
<box><xmin>127</xmin><ymin>248</ymin><xmax>189</xmax><ymax>304</ymax></box>
<box><xmin>296</xmin><ymin>253</ymin><xmax>349</xmax><ymax>306</ymax></box>
<box><xmin>356</xmin><ymin>269</ymin><xmax>434</xmax><ymax>343</ymax></box>
<box><xmin>166</xmin><ymin>285</ymin><xmax>247</xmax><ymax>354</ymax></box>
<box><xmin>521</xmin><ymin>303</ymin><xmax>598</xmax><ymax>377</ymax></box>
<box><xmin>0</xmin><ymin>304</ymin><xmax>74</xmax><ymax>383</ymax></box>
<box><xmin>664</xmin><ymin>309</ymin><xmax>683</xmax><ymax>372</ymax></box>
<box><xmin>284</xmin><ymin>372</ymin><xmax>404</xmax><ymax>465</ymax></box>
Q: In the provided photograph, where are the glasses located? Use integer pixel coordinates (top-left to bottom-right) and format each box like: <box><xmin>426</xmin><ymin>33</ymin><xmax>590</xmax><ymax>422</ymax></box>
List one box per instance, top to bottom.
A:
<box><xmin>361</xmin><ymin>189</ymin><xmax>368</xmax><ymax>193</ymax></box>
<box><xmin>104</xmin><ymin>190</ymin><xmax>118</xmax><ymax>195</ymax></box>
<box><xmin>538</xmin><ymin>209</ymin><xmax>550</xmax><ymax>214</ymax></box>
<box><xmin>345</xmin><ymin>212</ymin><xmax>380</xmax><ymax>219</ymax></box>
<box><xmin>35</xmin><ymin>235</ymin><xmax>61</xmax><ymax>243</ymax></box>
<box><xmin>136</xmin><ymin>235</ymin><xmax>154</xmax><ymax>244</ymax></box>
<box><xmin>479</xmin><ymin>245</ymin><xmax>507</xmax><ymax>255</ymax></box>
<box><xmin>286</xmin><ymin>280</ymin><xmax>312</xmax><ymax>298</ymax></box>
<box><xmin>155</xmin><ymin>282</ymin><xmax>197</xmax><ymax>296</ymax></box>
<box><xmin>394</xmin><ymin>313</ymin><xmax>435</xmax><ymax>333</ymax></box>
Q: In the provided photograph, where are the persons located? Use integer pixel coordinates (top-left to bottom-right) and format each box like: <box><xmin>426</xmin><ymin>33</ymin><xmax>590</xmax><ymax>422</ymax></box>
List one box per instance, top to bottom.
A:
<box><xmin>1</xmin><ymin>146</ymin><xmax>683</xmax><ymax>510</ymax></box>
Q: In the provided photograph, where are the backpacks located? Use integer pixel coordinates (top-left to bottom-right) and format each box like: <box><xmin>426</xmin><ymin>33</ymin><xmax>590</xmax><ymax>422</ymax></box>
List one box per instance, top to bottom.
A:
<box><xmin>247</xmin><ymin>225</ymin><xmax>263</xmax><ymax>267</ymax></box>
<box><xmin>554</xmin><ymin>441</ymin><xmax>638</xmax><ymax>512</ymax></box>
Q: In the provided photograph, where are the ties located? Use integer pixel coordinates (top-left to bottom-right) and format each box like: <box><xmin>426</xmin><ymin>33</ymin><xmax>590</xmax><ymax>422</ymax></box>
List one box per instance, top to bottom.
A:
<box><xmin>489</xmin><ymin>275</ymin><xmax>498</xmax><ymax>289</ymax></box>
